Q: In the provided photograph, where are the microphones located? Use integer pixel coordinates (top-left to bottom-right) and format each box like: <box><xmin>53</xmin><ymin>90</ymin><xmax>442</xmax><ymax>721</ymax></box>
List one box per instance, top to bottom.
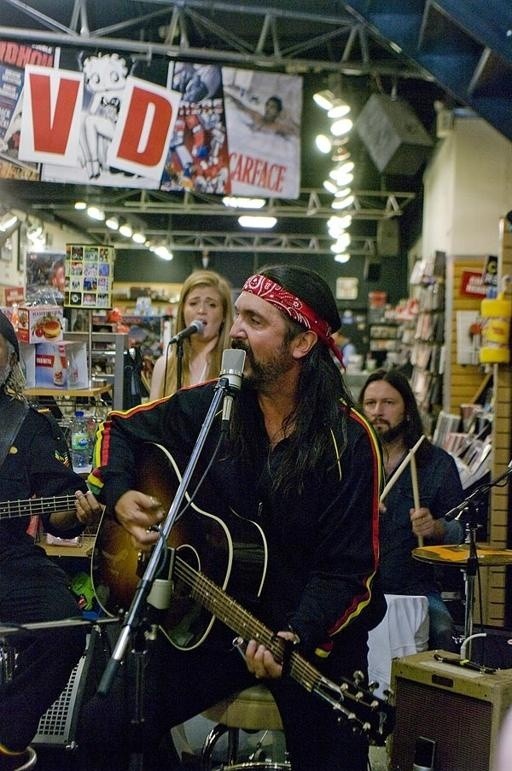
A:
<box><xmin>169</xmin><ymin>320</ymin><xmax>204</xmax><ymax>344</ymax></box>
<box><xmin>220</xmin><ymin>349</ymin><xmax>246</xmax><ymax>433</ymax></box>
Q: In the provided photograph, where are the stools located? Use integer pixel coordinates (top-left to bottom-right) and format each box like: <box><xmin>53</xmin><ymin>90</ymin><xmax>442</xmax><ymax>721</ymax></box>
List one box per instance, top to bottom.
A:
<box><xmin>200</xmin><ymin>681</ymin><xmax>290</xmax><ymax>771</ymax></box>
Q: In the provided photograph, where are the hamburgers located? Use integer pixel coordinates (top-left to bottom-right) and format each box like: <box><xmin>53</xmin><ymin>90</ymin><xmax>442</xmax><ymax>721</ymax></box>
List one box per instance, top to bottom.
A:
<box><xmin>43</xmin><ymin>321</ymin><xmax>60</xmax><ymax>337</ymax></box>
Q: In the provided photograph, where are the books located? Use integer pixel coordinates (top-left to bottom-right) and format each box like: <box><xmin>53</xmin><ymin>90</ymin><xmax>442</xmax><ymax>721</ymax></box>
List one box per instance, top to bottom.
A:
<box><xmin>406</xmin><ymin>259</ymin><xmax>493</xmax><ymax>489</ymax></box>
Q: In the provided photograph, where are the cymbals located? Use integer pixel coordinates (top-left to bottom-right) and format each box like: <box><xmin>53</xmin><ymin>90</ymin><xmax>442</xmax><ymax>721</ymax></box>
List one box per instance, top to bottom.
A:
<box><xmin>409</xmin><ymin>541</ymin><xmax>512</xmax><ymax>566</ymax></box>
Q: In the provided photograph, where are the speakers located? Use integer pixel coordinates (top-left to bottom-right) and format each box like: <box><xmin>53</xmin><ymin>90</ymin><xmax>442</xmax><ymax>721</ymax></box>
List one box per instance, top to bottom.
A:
<box><xmin>29</xmin><ymin>624</ymin><xmax>111</xmax><ymax>753</ymax></box>
<box><xmin>386</xmin><ymin>649</ymin><xmax>511</xmax><ymax>771</ymax></box>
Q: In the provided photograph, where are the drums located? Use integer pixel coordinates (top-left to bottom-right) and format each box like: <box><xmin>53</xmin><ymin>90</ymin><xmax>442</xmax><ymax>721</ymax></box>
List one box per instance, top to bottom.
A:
<box><xmin>368</xmin><ymin>592</ymin><xmax>431</xmax><ymax>698</ymax></box>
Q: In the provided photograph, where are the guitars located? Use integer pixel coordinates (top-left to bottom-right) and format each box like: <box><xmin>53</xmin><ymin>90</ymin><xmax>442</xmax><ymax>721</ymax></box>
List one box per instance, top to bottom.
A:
<box><xmin>91</xmin><ymin>440</ymin><xmax>395</xmax><ymax>747</ymax></box>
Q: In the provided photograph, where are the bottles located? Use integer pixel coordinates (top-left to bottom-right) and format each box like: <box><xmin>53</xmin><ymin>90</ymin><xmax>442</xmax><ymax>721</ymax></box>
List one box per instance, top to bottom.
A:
<box><xmin>52</xmin><ymin>344</ymin><xmax>69</xmax><ymax>385</ymax></box>
<box><xmin>67</xmin><ymin>350</ymin><xmax>78</xmax><ymax>385</ymax></box>
<box><xmin>69</xmin><ymin>410</ymin><xmax>92</xmax><ymax>474</ymax></box>
<box><xmin>465</xmin><ymin>522</ymin><xmax>476</xmax><ymax>544</ymax></box>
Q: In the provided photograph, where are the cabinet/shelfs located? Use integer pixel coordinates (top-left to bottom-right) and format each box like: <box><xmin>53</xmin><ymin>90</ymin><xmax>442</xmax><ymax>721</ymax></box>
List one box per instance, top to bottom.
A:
<box><xmin>25</xmin><ymin>379</ymin><xmax>115</xmax><ymax>562</ymax></box>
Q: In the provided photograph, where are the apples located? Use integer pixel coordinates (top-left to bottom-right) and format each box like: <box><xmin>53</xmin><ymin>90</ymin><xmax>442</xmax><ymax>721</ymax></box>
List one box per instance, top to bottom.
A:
<box><xmin>35</xmin><ymin>328</ymin><xmax>43</xmax><ymax>336</ymax></box>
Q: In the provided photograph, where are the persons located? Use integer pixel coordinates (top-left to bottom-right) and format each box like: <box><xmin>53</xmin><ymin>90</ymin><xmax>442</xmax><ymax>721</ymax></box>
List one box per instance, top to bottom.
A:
<box><xmin>76</xmin><ymin>266</ymin><xmax>387</xmax><ymax>771</ymax></box>
<box><xmin>150</xmin><ymin>270</ymin><xmax>234</xmax><ymax>400</ymax></box>
<box><xmin>0</xmin><ymin>309</ymin><xmax>106</xmax><ymax>771</ymax></box>
<box><xmin>359</xmin><ymin>370</ymin><xmax>468</xmax><ymax>655</ymax></box>
